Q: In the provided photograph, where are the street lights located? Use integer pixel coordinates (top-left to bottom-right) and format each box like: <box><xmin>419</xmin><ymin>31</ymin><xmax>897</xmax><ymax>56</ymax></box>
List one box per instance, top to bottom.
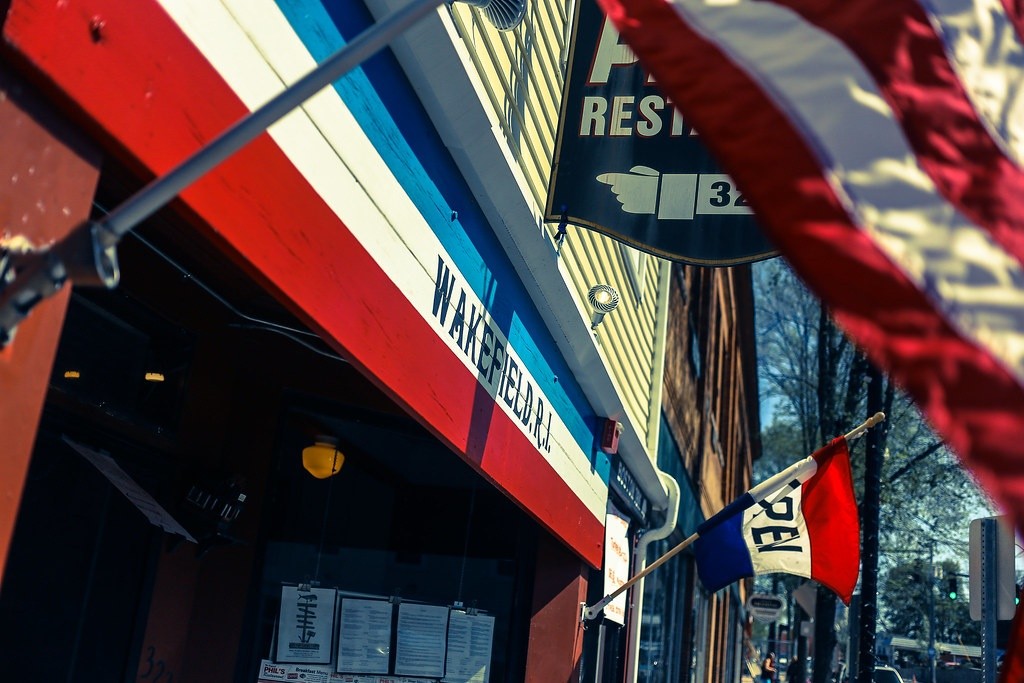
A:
<box><xmin>918</xmin><ymin>541</ymin><xmax>939</xmax><ymax>683</ymax></box>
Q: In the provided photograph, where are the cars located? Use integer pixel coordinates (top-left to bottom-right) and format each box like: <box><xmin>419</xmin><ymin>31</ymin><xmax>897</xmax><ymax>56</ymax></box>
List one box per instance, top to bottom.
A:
<box><xmin>873</xmin><ymin>666</ymin><xmax>903</xmax><ymax>683</ymax></box>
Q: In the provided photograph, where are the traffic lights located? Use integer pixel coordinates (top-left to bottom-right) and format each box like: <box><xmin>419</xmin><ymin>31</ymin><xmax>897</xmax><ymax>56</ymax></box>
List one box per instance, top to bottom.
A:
<box><xmin>949</xmin><ymin>577</ymin><xmax>958</xmax><ymax>601</ymax></box>
<box><xmin>1015</xmin><ymin>584</ymin><xmax>1022</xmax><ymax>607</ymax></box>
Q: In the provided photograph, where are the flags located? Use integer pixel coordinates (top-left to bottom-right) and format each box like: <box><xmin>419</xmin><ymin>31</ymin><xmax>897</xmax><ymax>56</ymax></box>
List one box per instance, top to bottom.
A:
<box><xmin>598</xmin><ymin>0</ymin><xmax>1024</xmax><ymax>540</ymax></box>
<box><xmin>696</xmin><ymin>437</ymin><xmax>861</xmax><ymax>607</ymax></box>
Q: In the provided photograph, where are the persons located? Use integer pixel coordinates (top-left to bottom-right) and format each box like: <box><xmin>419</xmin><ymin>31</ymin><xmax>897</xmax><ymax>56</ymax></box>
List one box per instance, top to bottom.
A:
<box><xmin>761</xmin><ymin>652</ymin><xmax>780</xmax><ymax>683</ymax></box>
<box><xmin>785</xmin><ymin>653</ymin><xmax>811</xmax><ymax>683</ymax></box>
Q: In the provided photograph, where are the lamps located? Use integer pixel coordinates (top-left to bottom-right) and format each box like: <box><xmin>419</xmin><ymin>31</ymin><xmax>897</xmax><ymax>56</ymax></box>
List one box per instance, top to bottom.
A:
<box><xmin>588</xmin><ymin>284</ymin><xmax>620</xmax><ymax>337</ymax></box>
<box><xmin>302</xmin><ymin>435</ymin><xmax>345</xmax><ymax>479</ymax></box>
